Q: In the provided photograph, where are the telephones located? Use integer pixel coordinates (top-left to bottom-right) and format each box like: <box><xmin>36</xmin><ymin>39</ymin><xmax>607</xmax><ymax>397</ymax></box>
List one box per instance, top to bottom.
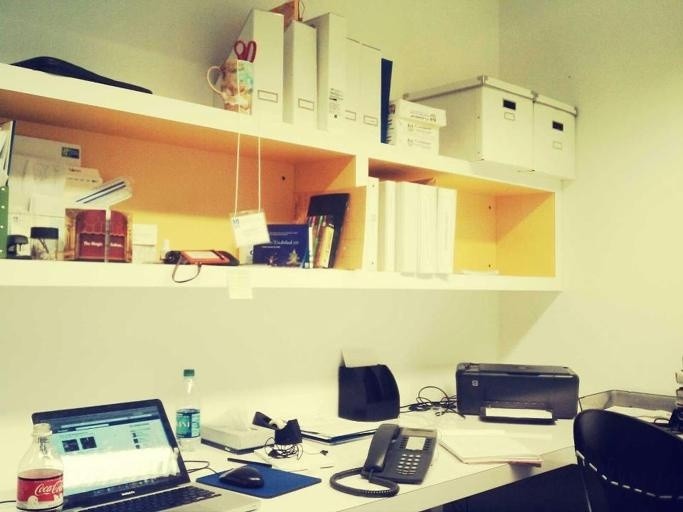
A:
<box><xmin>360</xmin><ymin>424</ymin><xmax>437</xmax><ymax>485</ymax></box>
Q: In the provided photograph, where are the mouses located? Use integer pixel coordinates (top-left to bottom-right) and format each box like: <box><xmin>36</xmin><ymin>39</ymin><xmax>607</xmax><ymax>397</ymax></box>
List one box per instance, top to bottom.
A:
<box><xmin>219</xmin><ymin>467</ymin><xmax>263</xmax><ymax>488</ymax></box>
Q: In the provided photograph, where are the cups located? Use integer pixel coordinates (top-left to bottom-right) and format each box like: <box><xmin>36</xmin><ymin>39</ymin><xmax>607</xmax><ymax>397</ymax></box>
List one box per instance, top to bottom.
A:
<box><xmin>206</xmin><ymin>59</ymin><xmax>253</xmax><ymax>115</ymax></box>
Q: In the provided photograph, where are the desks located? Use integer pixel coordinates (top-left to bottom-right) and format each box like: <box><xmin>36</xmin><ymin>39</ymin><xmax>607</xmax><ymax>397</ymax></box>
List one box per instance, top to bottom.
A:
<box><xmin>0</xmin><ymin>388</ymin><xmax>649</xmax><ymax>512</ymax></box>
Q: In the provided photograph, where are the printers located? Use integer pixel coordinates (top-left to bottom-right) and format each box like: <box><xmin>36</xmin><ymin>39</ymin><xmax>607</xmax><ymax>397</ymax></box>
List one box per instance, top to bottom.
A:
<box><xmin>456</xmin><ymin>363</ymin><xmax>580</xmax><ymax>424</ymax></box>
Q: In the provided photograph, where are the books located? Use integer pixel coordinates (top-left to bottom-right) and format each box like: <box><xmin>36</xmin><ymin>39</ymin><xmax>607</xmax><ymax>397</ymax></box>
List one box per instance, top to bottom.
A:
<box><xmin>366</xmin><ymin>176</ymin><xmax>457</xmax><ymax>274</ymax></box>
<box><xmin>438</xmin><ymin>431</ymin><xmax>544</xmax><ymax>464</ymax></box>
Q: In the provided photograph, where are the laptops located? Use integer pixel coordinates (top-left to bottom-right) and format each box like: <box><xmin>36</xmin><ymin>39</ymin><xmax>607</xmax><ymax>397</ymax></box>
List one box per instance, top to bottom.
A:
<box><xmin>32</xmin><ymin>399</ymin><xmax>262</xmax><ymax>512</ymax></box>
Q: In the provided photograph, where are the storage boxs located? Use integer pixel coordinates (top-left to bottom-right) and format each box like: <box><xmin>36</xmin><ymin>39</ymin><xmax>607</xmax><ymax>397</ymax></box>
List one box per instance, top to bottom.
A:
<box><xmin>395</xmin><ymin>75</ymin><xmax>534</xmax><ymax>169</ymax></box>
<box><xmin>533</xmin><ymin>94</ymin><xmax>577</xmax><ymax>181</ymax></box>
<box><xmin>386</xmin><ymin>100</ymin><xmax>447</xmax><ymax>156</ymax></box>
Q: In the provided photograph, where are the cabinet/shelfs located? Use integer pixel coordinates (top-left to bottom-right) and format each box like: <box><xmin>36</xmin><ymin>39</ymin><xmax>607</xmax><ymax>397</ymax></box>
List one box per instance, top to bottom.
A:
<box><xmin>0</xmin><ymin>63</ymin><xmax>562</xmax><ymax>293</ymax></box>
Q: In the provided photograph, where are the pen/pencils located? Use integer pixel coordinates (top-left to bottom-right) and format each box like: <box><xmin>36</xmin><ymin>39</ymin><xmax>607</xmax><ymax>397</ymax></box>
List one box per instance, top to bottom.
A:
<box><xmin>302</xmin><ymin>215</ymin><xmax>335</xmax><ymax>269</ymax></box>
<box><xmin>228</xmin><ymin>457</ymin><xmax>272</xmax><ymax>468</ymax></box>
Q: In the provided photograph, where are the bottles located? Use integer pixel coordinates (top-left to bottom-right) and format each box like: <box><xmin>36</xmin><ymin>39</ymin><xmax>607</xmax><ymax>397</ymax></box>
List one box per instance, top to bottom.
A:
<box><xmin>30</xmin><ymin>227</ymin><xmax>59</xmax><ymax>261</ymax></box>
<box><xmin>175</xmin><ymin>369</ymin><xmax>200</xmax><ymax>455</ymax></box>
<box><xmin>17</xmin><ymin>423</ymin><xmax>64</xmax><ymax>511</ymax></box>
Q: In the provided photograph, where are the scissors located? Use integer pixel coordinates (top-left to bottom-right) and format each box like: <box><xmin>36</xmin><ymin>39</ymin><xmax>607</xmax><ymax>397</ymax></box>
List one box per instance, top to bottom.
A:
<box><xmin>234</xmin><ymin>40</ymin><xmax>257</xmax><ymax>64</ymax></box>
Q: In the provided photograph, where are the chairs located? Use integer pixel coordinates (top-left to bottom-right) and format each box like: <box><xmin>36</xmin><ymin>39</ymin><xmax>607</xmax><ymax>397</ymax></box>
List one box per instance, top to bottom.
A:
<box><xmin>574</xmin><ymin>409</ymin><xmax>683</xmax><ymax>512</ymax></box>
